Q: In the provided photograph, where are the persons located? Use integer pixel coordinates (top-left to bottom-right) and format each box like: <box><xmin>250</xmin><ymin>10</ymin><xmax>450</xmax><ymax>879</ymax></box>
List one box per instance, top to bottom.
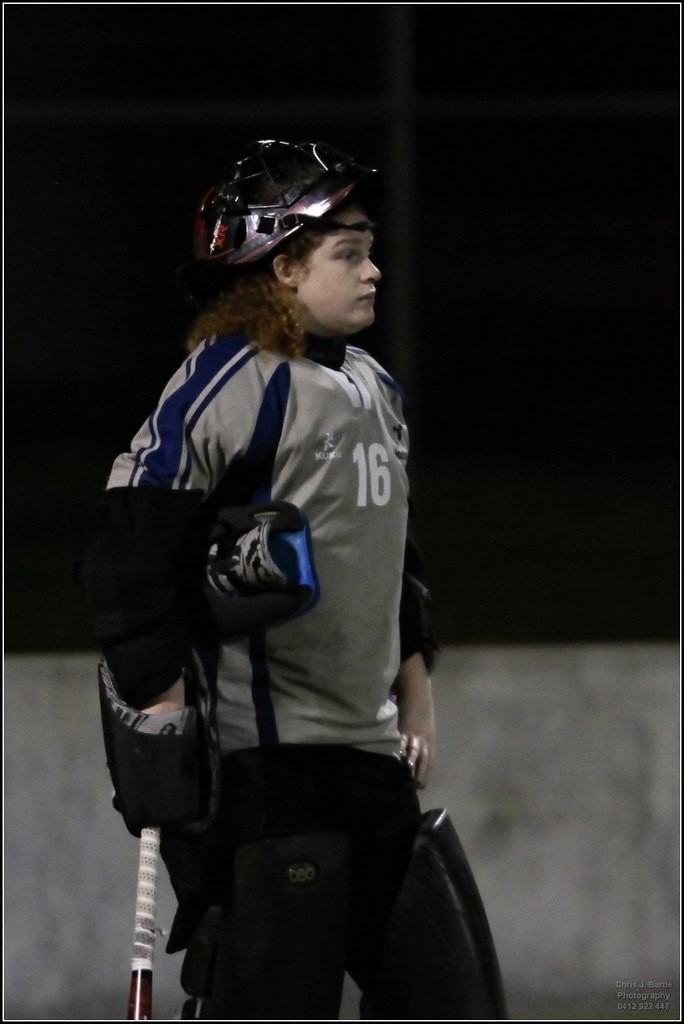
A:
<box><xmin>100</xmin><ymin>128</ymin><xmax>511</xmax><ymax>1024</ymax></box>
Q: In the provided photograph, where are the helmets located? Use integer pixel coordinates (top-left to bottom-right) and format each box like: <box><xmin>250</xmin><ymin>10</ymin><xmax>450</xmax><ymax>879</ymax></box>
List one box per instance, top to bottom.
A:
<box><xmin>188</xmin><ymin>135</ymin><xmax>372</xmax><ymax>282</ymax></box>
